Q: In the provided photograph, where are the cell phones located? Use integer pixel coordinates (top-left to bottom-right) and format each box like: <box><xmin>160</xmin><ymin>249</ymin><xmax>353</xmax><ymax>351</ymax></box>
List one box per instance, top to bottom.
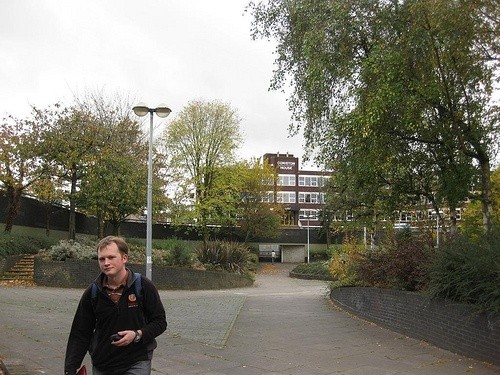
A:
<box><xmin>111</xmin><ymin>334</ymin><xmax>122</xmax><ymax>341</ymax></box>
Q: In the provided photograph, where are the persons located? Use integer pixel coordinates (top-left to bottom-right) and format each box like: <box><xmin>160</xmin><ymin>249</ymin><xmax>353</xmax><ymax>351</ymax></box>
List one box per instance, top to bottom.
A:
<box><xmin>64</xmin><ymin>236</ymin><xmax>167</xmax><ymax>375</ymax></box>
<box><xmin>272</xmin><ymin>250</ymin><xmax>276</xmax><ymax>265</ymax></box>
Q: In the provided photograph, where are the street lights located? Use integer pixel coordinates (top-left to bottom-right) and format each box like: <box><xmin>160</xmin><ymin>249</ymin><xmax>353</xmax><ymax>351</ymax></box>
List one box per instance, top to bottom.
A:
<box><xmin>131</xmin><ymin>100</ymin><xmax>173</xmax><ymax>285</ymax></box>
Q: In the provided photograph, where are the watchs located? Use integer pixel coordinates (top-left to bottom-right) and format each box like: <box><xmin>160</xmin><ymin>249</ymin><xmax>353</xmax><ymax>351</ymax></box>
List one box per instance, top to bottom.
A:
<box><xmin>134</xmin><ymin>330</ymin><xmax>140</xmax><ymax>343</ymax></box>
